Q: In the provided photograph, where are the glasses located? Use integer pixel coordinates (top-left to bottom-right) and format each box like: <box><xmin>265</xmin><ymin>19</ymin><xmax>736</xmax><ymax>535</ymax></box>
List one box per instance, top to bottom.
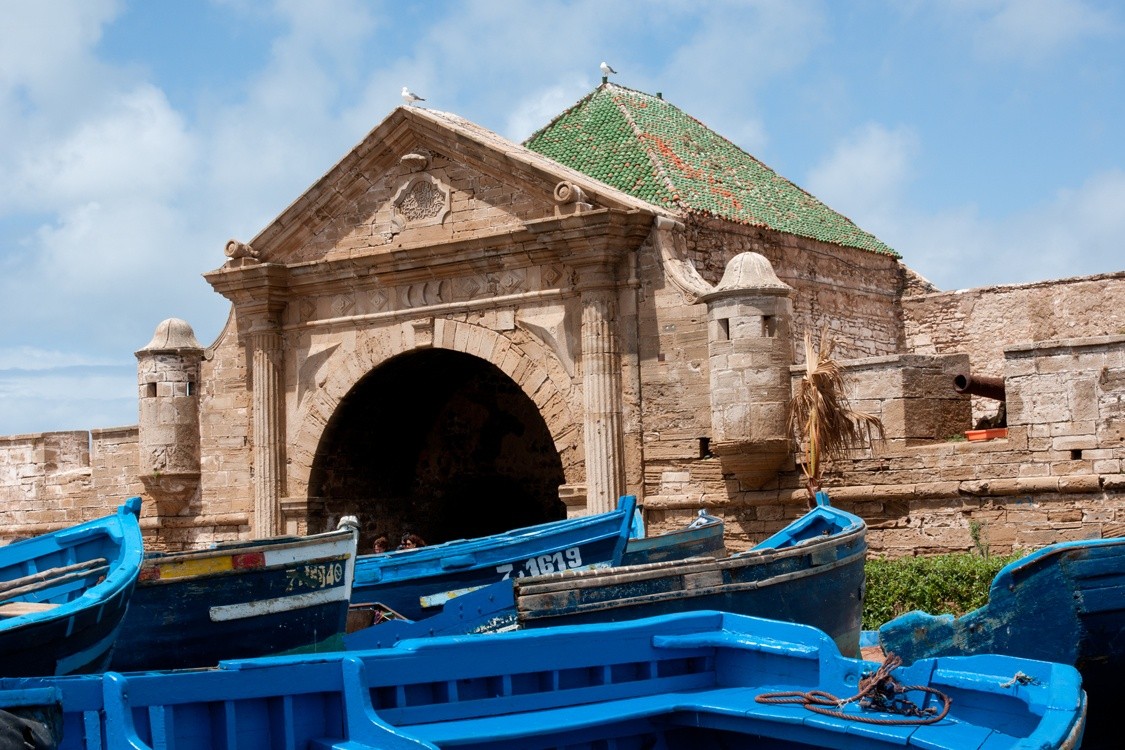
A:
<box><xmin>405</xmin><ymin>543</ymin><xmax>411</xmax><ymax>546</ymax></box>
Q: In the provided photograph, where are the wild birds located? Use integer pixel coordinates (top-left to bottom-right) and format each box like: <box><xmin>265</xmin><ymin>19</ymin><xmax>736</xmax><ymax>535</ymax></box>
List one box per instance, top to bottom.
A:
<box><xmin>600</xmin><ymin>62</ymin><xmax>618</xmax><ymax>77</ymax></box>
<box><xmin>400</xmin><ymin>87</ymin><xmax>428</xmax><ymax>105</ymax></box>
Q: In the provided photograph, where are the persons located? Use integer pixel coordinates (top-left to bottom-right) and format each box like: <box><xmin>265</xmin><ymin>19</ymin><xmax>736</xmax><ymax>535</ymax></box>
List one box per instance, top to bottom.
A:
<box><xmin>398</xmin><ymin>532</ymin><xmax>425</xmax><ymax>552</ymax></box>
<box><xmin>375</xmin><ymin>538</ymin><xmax>388</xmax><ymax>554</ymax></box>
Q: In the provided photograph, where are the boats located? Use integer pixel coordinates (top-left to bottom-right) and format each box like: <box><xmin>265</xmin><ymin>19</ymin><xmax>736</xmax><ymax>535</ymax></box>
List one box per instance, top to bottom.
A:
<box><xmin>510</xmin><ymin>488</ymin><xmax>870</xmax><ymax>659</ymax></box>
<box><xmin>346</xmin><ymin>492</ymin><xmax>640</xmax><ymax>638</ymax></box>
<box><xmin>0</xmin><ymin>495</ymin><xmax>146</xmax><ymax>678</ymax></box>
<box><xmin>620</xmin><ymin>508</ymin><xmax>728</xmax><ymax>568</ymax></box>
<box><xmin>104</xmin><ymin>513</ymin><xmax>362</xmax><ymax>672</ymax></box>
<box><xmin>876</xmin><ymin>534</ymin><xmax>1125</xmax><ymax>710</ymax></box>
<box><xmin>0</xmin><ymin>610</ymin><xmax>1089</xmax><ymax>750</ymax></box>
